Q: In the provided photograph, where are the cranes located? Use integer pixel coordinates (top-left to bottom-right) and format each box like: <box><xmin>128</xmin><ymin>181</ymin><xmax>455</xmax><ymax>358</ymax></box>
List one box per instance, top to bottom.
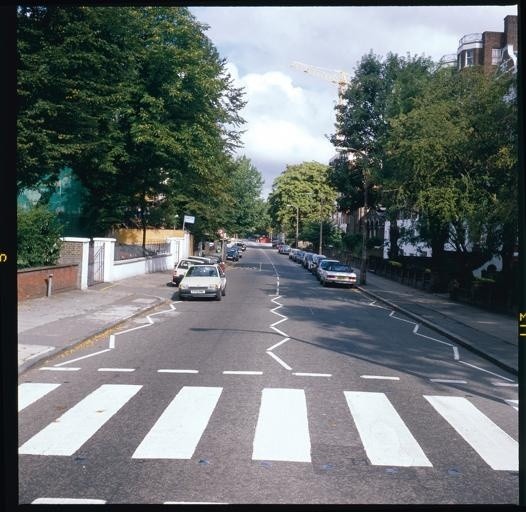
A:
<box><xmin>286</xmin><ymin>58</ymin><xmax>355</xmax><ymax>161</ymax></box>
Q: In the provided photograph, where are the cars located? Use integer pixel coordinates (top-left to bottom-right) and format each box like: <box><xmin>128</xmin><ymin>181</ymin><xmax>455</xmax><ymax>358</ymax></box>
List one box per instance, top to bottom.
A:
<box><xmin>250</xmin><ymin>233</ymin><xmax>267</xmax><ymax>242</ymax></box>
<box><xmin>171</xmin><ymin>235</ymin><xmax>247</xmax><ymax>283</ymax></box>
<box><xmin>271</xmin><ymin>238</ymin><xmax>359</xmax><ymax>287</ymax></box>
<box><xmin>176</xmin><ymin>264</ymin><xmax>228</xmax><ymax>302</ymax></box>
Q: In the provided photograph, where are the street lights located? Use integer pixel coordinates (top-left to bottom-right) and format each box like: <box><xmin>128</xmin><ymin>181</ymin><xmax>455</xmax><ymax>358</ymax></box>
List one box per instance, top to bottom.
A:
<box><xmin>333</xmin><ymin>144</ymin><xmax>367</xmax><ymax>289</ymax></box>
<box><xmin>302</xmin><ymin>191</ymin><xmax>323</xmax><ymax>254</ymax></box>
<box><xmin>287</xmin><ymin>204</ymin><xmax>299</xmax><ymax>249</ymax></box>
